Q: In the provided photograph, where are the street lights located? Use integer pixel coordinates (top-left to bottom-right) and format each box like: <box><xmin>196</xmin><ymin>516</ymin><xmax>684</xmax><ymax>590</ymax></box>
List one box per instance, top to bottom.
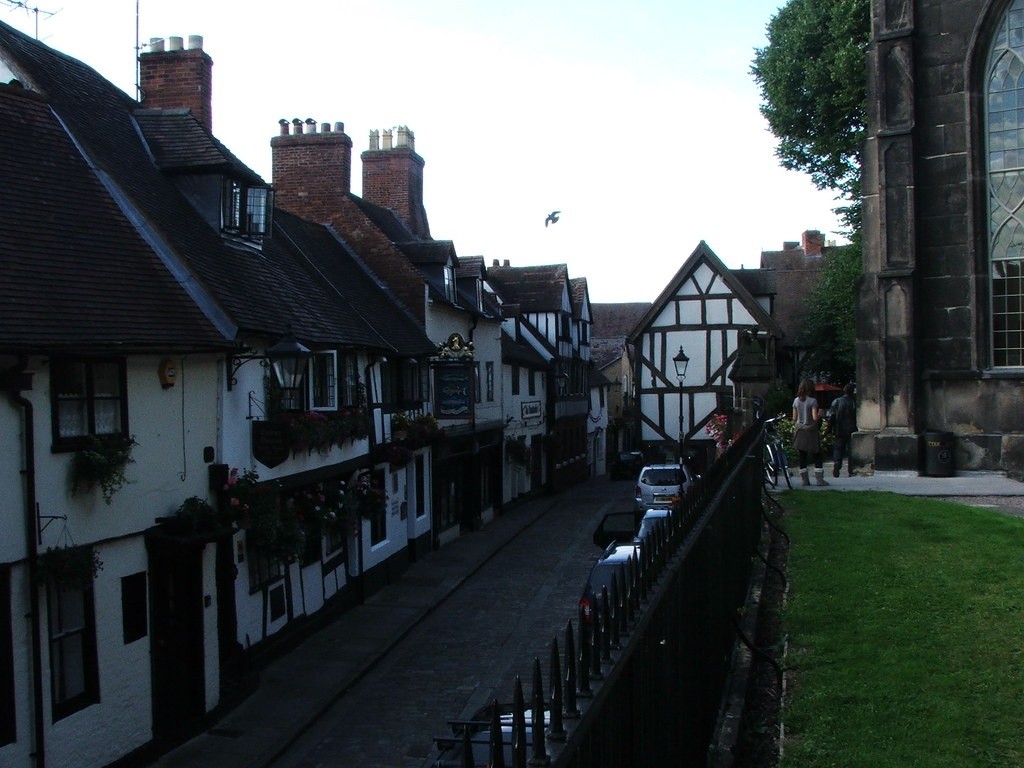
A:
<box><xmin>673</xmin><ymin>345</ymin><xmax>690</xmax><ymax>465</ymax></box>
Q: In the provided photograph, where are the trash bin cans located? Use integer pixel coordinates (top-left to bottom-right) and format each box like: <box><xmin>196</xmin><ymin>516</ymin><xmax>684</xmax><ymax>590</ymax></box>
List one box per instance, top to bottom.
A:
<box><xmin>925</xmin><ymin>431</ymin><xmax>953</xmax><ymax>478</ymax></box>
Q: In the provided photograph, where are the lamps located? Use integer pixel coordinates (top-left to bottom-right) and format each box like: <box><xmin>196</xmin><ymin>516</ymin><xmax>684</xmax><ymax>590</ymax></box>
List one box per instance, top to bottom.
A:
<box><xmin>225</xmin><ymin>321</ymin><xmax>315</xmax><ymax>417</ymax></box>
<box><xmin>541</xmin><ymin>366</ymin><xmax>569</xmax><ymax>389</ymax></box>
<box><xmin>606</xmin><ymin>376</ymin><xmax>622</xmax><ymax>392</ymax></box>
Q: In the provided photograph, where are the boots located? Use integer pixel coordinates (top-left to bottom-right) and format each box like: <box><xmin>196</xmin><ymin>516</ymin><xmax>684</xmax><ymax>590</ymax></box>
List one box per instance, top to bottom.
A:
<box><xmin>799</xmin><ymin>468</ymin><xmax>811</xmax><ymax>486</ymax></box>
<box><xmin>815</xmin><ymin>468</ymin><xmax>829</xmax><ymax>486</ymax></box>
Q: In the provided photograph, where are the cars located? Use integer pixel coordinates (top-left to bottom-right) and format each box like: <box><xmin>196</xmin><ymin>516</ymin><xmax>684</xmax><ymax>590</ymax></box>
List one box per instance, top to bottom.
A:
<box><xmin>593</xmin><ymin>505</ymin><xmax>676</xmax><ymax>544</ymax></box>
<box><xmin>432</xmin><ymin>701</ymin><xmax>557</xmax><ymax>767</ymax></box>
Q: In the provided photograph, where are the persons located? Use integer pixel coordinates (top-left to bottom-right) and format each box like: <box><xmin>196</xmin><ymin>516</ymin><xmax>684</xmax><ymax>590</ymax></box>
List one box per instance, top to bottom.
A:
<box><xmin>791</xmin><ymin>378</ymin><xmax>828</xmax><ymax>488</ymax></box>
<box><xmin>827</xmin><ymin>382</ymin><xmax>867</xmax><ymax>477</ymax></box>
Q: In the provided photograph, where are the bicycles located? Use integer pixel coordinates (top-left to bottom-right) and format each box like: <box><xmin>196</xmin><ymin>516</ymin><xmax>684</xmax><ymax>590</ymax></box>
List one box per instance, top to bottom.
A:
<box><xmin>757</xmin><ymin>411</ymin><xmax>793</xmax><ymax>489</ymax></box>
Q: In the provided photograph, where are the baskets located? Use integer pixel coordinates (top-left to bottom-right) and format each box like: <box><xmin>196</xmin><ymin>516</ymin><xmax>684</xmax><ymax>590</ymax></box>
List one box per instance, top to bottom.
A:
<box><xmin>46</xmin><ymin>526</ymin><xmax>104</xmax><ymax>604</ymax></box>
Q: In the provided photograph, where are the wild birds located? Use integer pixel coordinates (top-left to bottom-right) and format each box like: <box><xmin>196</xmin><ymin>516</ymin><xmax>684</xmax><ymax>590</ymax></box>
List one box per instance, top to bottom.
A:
<box><xmin>546</xmin><ymin>212</ymin><xmax>559</xmax><ymax>227</ymax></box>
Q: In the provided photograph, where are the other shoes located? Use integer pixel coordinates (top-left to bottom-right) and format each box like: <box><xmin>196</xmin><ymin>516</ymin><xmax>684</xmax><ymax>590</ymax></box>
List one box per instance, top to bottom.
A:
<box><xmin>833</xmin><ymin>461</ymin><xmax>842</xmax><ymax>477</ymax></box>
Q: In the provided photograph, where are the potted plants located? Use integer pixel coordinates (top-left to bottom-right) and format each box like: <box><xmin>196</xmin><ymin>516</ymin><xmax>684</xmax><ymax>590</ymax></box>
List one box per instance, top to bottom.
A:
<box><xmin>391</xmin><ymin>413</ymin><xmax>412</xmax><ymax>439</ymax></box>
<box><xmin>67</xmin><ymin>430</ymin><xmax>141</xmax><ymax>504</ymax></box>
<box><xmin>35</xmin><ymin>543</ymin><xmax>103</xmax><ymax>595</ymax></box>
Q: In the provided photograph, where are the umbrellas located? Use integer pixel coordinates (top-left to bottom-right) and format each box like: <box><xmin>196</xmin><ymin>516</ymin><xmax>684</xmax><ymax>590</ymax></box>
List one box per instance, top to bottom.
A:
<box><xmin>813</xmin><ymin>382</ymin><xmax>841</xmax><ymax>391</ymax></box>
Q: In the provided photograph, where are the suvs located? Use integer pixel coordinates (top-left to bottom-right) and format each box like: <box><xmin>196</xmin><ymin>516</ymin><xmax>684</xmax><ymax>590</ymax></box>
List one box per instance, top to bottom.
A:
<box><xmin>633</xmin><ymin>462</ymin><xmax>702</xmax><ymax>513</ymax></box>
<box><xmin>579</xmin><ymin>538</ymin><xmax>646</xmax><ymax>642</ymax></box>
